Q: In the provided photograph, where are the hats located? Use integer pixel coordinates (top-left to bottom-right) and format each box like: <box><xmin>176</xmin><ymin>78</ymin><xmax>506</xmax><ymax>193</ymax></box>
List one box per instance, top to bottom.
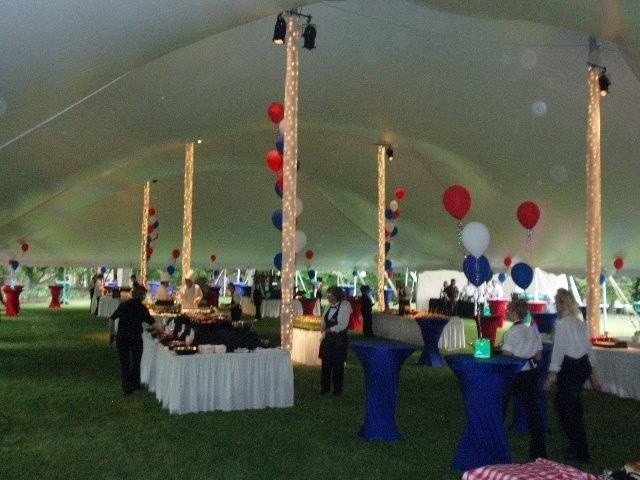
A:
<box><xmin>185</xmin><ymin>271</ymin><xmax>195</xmax><ymax>281</ymax></box>
<box><xmin>160</xmin><ymin>272</ymin><xmax>169</xmax><ymax>282</ymax></box>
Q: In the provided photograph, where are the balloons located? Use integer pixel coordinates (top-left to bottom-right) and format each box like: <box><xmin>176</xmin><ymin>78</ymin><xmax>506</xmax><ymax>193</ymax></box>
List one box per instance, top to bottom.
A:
<box><xmin>267</xmin><ymin>102</ymin><xmax>307</xmax><ymax>269</ymax></box>
<box><xmin>444</xmin><ymin>185</ymin><xmax>490</xmax><ymax>286</ymax></box>
<box><xmin>384</xmin><ymin>188</ymin><xmax>404</xmax><ymax>271</ymax></box>
<box><xmin>517</xmin><ymin>201</ymin><xmax>540</xmax><ymax>229</ymax></box>
<box><xmin>146</xmin><ymin>209</ymin><xmax>159</xmax><ymax>263</ymax></box>
<box><xmin>11</xmin><ymin>244</ymin><xmax>28</xmax><ymax>270</ymax></box>
<box><xmin>167</xmin><ymin>249</ymin><xmax>180</xmax><ymax>272</ymax></box>
<box><xmin>306</xmin><ymin>250</ymin><xmax>313</xmax><ymax>259</ymax></box>
<box><xmin>210</xmin><ymin>255</ymin><xmax>216</xmax><ymax>260</ymax></box>
<box><xmin>498</xmin><ymin>258</ymin><xmax>532</xmax><ymax>289</ymax></box>
<box><xmin>614</xmin><ymin>258</ymin><xmax>622</xmax><ymax>270</ymax></box>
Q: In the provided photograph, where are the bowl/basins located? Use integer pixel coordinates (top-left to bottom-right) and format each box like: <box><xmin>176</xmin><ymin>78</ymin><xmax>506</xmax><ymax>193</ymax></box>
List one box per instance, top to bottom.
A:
<box><xmin>216</xmin><ymin>313</ymin><xmax>253</xmax><ymax>330</ymax></box>
<box><xmin>197</xmin><ymin>344</ymin><xmax>215</xmax><ymax>354</ymax></box>
<box><xmin>213</xmin><ymin>344</ymin><xmax>228</xmax><ymax>354</ymax></box>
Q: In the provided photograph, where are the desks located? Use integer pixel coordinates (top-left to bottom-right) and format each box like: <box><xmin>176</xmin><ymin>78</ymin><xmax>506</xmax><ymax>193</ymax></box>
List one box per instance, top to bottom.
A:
<box><xmin>89</xmin><ymin>293</ymin><xmax>466</xmax><ymax>412</ymax></box>
<box><xmin>588</xmin><ymin>345</ymin><xmax>640</xmax><ymax>400</ymax></box>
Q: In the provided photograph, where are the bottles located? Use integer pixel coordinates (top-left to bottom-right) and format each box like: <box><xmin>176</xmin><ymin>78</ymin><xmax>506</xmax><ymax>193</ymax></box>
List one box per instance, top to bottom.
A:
<box><xmin>292</xmin><ymin>313</ymin><xmax>322</xmax><ymax>331</ymax></box>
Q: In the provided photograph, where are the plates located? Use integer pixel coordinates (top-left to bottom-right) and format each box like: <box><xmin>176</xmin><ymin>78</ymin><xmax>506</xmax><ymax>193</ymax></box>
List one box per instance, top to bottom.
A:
<box><xmin>144</xmin><ymin>324</ymin><xmax>199</xmax><ymax>355</ymax></box>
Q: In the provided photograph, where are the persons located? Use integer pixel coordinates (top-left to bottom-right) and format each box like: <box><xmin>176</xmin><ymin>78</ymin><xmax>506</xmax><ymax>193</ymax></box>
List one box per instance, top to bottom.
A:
<box><xmin>90</xmin><ymin>274</ymin><xmax>458</xmax><ymax>323</ymax></box>
<box><xmin>360</xmin><ymin>285</ymin><xmax>374</xmax><ymax>336</ymax></box>
<box><xmin>108</xmin><ymin>286</ymin><xmax>171</xmax><ymax>393</ymax></box>
<box><xmin>317</xmin><ymin>286</ymin><xmax>353</xmax><ymax>397</ymax></box>
<box><xmin>501</xmin><ymin>288</ymin><xmax>601</xmax><ymax>460</ymax></box>
